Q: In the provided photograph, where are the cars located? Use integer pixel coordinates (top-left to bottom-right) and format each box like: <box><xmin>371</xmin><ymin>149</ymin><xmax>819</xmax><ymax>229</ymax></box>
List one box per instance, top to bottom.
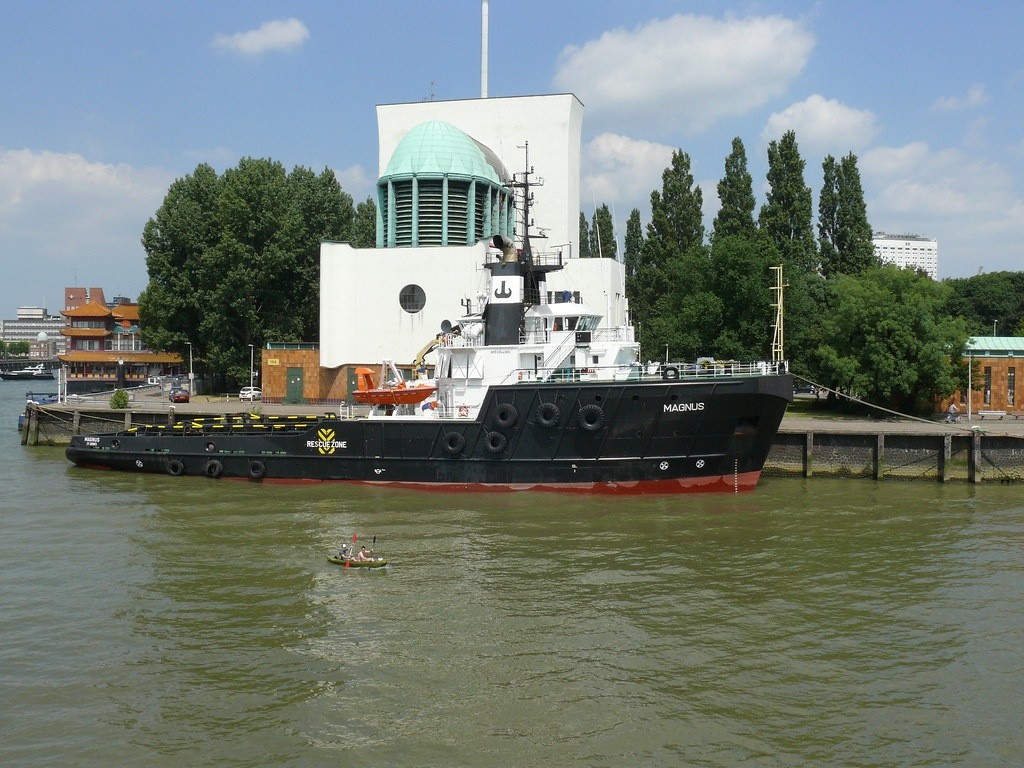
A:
<box><xmin>792</xmin><ymin>383</ymin><xmax>816</xmax><ymax>395</ymax></box>
<box><xmin>169</xmin><ymin>386</ymin><xmax>183</xmax><ymax>400</ymax></box>
<box><xmin>239</xmin><ymin>387</ymin><xmax>262</xmax><ymax>401</ymax></box>
<box><xmin>170</xmin><ymin>390</ymin><xmax>190</xmax><ymax>403</ymax></box>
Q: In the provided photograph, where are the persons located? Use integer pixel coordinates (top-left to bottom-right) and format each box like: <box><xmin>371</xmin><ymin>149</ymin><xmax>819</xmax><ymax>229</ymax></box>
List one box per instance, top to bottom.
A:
<box><xmin>948</xmin><ymin>403</ymin><xmax>960</xmax><ymax>419</ymax></box>
<box><xmin>336</xmin><ymin>543</ymin><xmax>357</xmax><ymax>562</ymax></box>
<box><xmin>356</xmin><ymin>546</ymin><xmax>375</xmax><ymax>562</ymax></box>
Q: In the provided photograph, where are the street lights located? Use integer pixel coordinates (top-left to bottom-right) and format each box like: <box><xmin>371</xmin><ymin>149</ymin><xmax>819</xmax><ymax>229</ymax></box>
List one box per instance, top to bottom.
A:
<box><xmin>184</xmin><ymin>341</ymin><xmax>195</xmax><ymax>396</ymax></box>
<box><xmin>247</xmin><ymin>343</ymin><xmax>254</xmax><ymax>403</ymax></box>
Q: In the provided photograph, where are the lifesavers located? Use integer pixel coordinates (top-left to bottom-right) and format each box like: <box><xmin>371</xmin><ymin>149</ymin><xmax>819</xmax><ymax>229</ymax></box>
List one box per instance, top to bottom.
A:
<box><xmin>459</xmin><ymin>406</ymin><xmax>468</xmax><ymax>417</ymax></box>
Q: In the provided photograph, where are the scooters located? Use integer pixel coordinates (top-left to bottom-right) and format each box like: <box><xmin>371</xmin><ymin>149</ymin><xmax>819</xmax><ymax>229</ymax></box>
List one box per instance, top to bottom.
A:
<box><xmin>946</xmin><ymin>411</ymin><xmax>964</xmax><ymax>424</ymax></box>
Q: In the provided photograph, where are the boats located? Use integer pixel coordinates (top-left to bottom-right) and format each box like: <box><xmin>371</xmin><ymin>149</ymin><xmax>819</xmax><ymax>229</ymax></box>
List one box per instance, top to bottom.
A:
<box><xmin>59</xmin><ymin>140</ymin><xmax>797</xmax><ymax>499</ymax></box>
<box><xmin>0</xmin><ymin>362</ymin><xmax>56</xmax><ymax>381</ymax></box>
<box><xmin>18</xmin><ymin>392</ymin><xmax>81</xmax><ymax>433</ymax></box>
<box><xmin>326</xmin><ymin>554</ymin><xmax>388</xmax><ymax>569</ymax></box>
